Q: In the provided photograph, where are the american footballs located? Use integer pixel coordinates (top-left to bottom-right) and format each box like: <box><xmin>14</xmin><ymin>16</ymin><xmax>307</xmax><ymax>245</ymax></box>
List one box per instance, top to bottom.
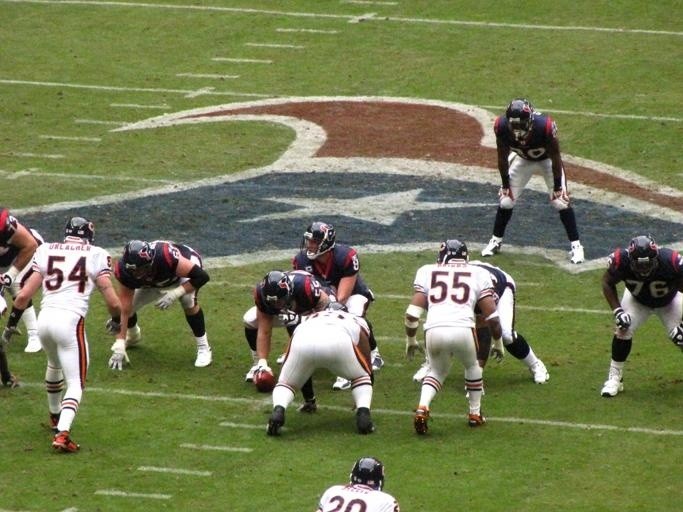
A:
<box><xmin>253</xmin><ymin>371</ymin><xmax>273</xmax><ymax>391</ymax></box>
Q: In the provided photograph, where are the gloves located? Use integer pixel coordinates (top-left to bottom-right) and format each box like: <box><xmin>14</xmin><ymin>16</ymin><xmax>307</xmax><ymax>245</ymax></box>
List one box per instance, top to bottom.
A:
<box><xmin>498</xmin><ymin>187</ymin><xmax>516</xmax><ymax>202</ymax></box>
<box><xmin>551</xmin><ymin>190</ymin><xmax>569</xmax><ymax>205</ymax></box>
<box><xmin>0</xmin><ymin>264</ymin><xmax>19</xmax><ymax>289</ymax></box>
<box><xmin>153</xmin><ymin>284</ymin><xmax>186</xmax><ymax>310</ymax></box>
<box><xmin>615</xmin><ymin>309</ymin><xmax>631</xmax><ymax>329</ymax></box>
<box><xmin>279</xmin><ymin>312</ymin><xmax>300</xmax><ymax>326</ymax></box>
<box><xmin>669</xmin><ymin>324</ymin><xmax>682</xmax><ymax>346</ymax></box>
<box><xmin>2</xmin><ymin>327</ymin><xmax>22</xmax><ymax>344</ymax></box>
<box><xmin>405</xmin><ymin>337</ymin><xmax>424</xmax><ymax>360</ymax></box>
<box><xmin>108</xmin><ymin>339</ymin><xmax>131</xmax><ymax>372</ymax></box>
<box><xmin>491</xmin><ymin>341</ymin><xmax>505</xmax><ymax>364</ymax></box>
<box><xmin>249</xmin><ymin>360</ymin><xmax>274</xmax><ymax>384</ymax></box>
<box><xmin>296</xmin><ymin>401</ymin><xmax>317</xmax><ymax>413</ymax></box>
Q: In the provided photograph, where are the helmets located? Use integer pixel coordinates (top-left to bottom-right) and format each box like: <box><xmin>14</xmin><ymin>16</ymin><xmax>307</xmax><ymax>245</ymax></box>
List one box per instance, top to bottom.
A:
<box><xmin>505</xmin><ymin>98</ymin><xmax>534</xmax><ymax>135</ymax></box>
<box><xmin>65</xmin><ymin>217</ymin><xmax>95</xmax><ymax>242</ymax></box>
<box><xmin>303</xmin><ymin>220</ymin><xmax>335</xmax><ymax>260</ymax></box>
<box><xmin>630</xmin><ymin>236</ymin><xmax>659</xmax><ymax>271</ymax></box>
<box><xmin>438</xmin><ymin>239</ymin><xmax>468</xmax><ymax>263</ymax></box>
<box><xmin>122</xmin><ymin>241</ymin><xmax>152</xmax><ymax>271</ymax></box>
<box><xmin>261</xmin><ymin>270</ymin><xmax>290</xmax><ymax>301</ymax></box>
<box><xmin>325</xmin><ymin>302</ymin><xmax>347</xmax><ymax>310</ymax></box>
<box><xmin>350</xmin><ymin>456</ymin><xmax>385</xmax><ymax>491</ymax></box>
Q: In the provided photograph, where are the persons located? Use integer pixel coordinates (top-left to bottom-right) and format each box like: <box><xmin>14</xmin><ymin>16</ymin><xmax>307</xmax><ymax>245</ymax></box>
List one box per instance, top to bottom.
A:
<box><xmin>479</xmin><ymin>99</ymin><xmax>584</xmax><ymax>264</ymax></box>
<box><xmin>0</xmin><ymin>205</ymin><xmax>211</xmax><ymax>452</ymax></box>
<box><xmin>244</xmin><ymin>222</ymin><xmax>384</xmax><ymax>434</ymax></box>
<box><xmin>600</xmin><ymin>234</ymin><xmax>682</xmax><ymax>398</ymax></box>
<box><xmin>406</xmin><ymin>239</ymin><xmax>550</xmax><ymax>435</ymax></box>
<box><xmin>317</xmin><ymin>457</ymin><xmax>400</xmax><ymax>512</ymax></box>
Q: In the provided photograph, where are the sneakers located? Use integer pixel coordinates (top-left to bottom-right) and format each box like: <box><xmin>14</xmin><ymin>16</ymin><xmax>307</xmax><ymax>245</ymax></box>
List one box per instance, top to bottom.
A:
<box><xmin>481</xmin><ymin>238</ymin><xmax>503</xmax><ymax>256</ymax></box>
<box><xmin>570</xmin><ymin>242</ymin><xmax>584</xmax><ymax>264</ymax></box>
<box><xmin>126</xmin><ymin>333</ymin><xmax>142</xmax><ymax>348</ymax></box>
<box><xmin>24</xmin><ymin>335</ymin><xmax>43</xmax><ymax>355</ymax></box>
<box><xmin>194</xmin><ymin>346</ymin><xmax>213</xmax><ymax>367</ymax></box>
<box><xmin>276</xmin><ymin>353</ymin><xmax>287</xmax><ymax>363</ymax></box>
<box><xmin>370</xmin><ymin>354</ymin><xmax>384</xmax><ymax>370</ymax></box>
<box><xmin>530</xmin><ymin>359</ymin><xmax>549</xmax><ymax>385</ymax></box>
<box><xmin>245</xmin><ymin>364</ymin><xmax>255</xmax><ymax>383</ymax></box>
<box><xmin>1</xmin><ymin>373</ymin><xmax>19</xmax><ymax>389</ymax></box>
<box><xmin>331</xmin><ymin>375</ymin><xmax>351</xmax><ymax>391</ymax></box>
<box><xmin>601</xmin><ymin>375</ymin><xmax>624</xmax><ymax>397</ymax></box>
<box><xmin>264</xmin><ymin>408</ymin><xmax>285</xmax><ymax>437</ymax></box>
<box><xmin>355</xmin><ymin>408</ymin><xmax>376</xmax><ymax>433</ymax></box>
<box><xmin>415</xmin><ymin>408</ymin><xmax>428</xmax><ymax>434</ymax></box>
<box><xmin>49</xmin><ymin>413</ymin><xmax>59</xmax><ymax>430</ymax></box>
<box><xmin>469</xmin><ymin>413</ymin><xmax>485</xmax><ymax>428</ymax></box>
<box><xmin>53</xmin><ymin>430</ymin><xmax>80</xmax><ymax>449</ymax></box>
